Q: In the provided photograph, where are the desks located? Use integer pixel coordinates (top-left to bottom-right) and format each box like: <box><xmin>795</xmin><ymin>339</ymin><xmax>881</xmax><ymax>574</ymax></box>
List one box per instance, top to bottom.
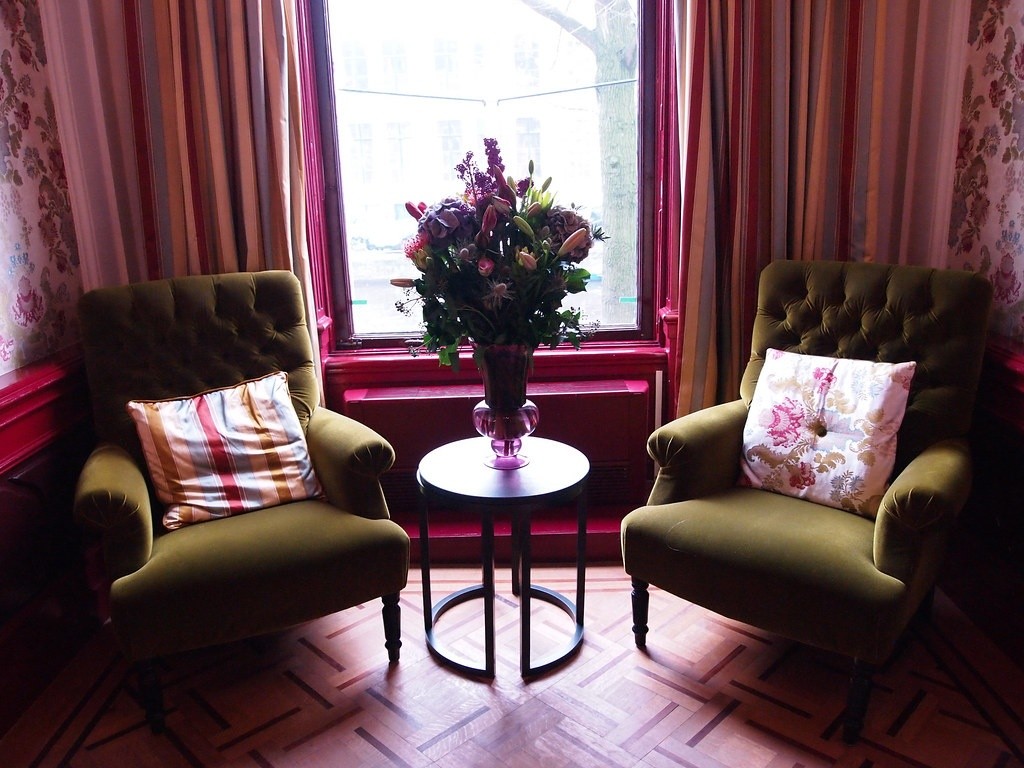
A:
<box><xmin>416</xmin><ymin>436</ymin><xmax>591</xmax><ymax>678</ymax></box>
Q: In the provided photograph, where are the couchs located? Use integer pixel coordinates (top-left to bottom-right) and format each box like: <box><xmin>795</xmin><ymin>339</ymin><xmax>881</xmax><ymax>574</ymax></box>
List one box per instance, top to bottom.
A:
<box><xmin>620</xmin><ymin>259</ymin><xmax>994</xmax><ymax>746</ymax></box>
<box><xmin>71</xmin><ymin>270</ymin><xmax>410</xmax><ymax>735</ymax></box>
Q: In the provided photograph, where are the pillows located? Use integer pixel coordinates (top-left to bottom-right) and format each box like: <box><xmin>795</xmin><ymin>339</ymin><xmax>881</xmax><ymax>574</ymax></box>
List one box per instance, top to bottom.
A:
<box><xmin>733</xmin><ymin>348</ymin><xmax>916</xmax><ymax>519</ymax></box>
<box><xmin>126</xmin><ymin>371</ymin><xmax>328</xmax><ymax>530</ymax></box>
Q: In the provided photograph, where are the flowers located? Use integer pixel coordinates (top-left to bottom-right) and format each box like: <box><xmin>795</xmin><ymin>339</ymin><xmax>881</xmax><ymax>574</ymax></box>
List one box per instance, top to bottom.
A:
<box><xmin>390</xmin><ymin>137</ymin><xmax>607</xmax><ymax>453</ymax></box>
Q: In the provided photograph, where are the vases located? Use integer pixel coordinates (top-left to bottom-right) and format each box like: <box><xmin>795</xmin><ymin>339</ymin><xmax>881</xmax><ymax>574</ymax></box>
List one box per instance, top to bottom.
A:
<box><xmin>469</xmin><ymin>341</ymin><xmax>539</xmax><ymax>469</ymax></box>
